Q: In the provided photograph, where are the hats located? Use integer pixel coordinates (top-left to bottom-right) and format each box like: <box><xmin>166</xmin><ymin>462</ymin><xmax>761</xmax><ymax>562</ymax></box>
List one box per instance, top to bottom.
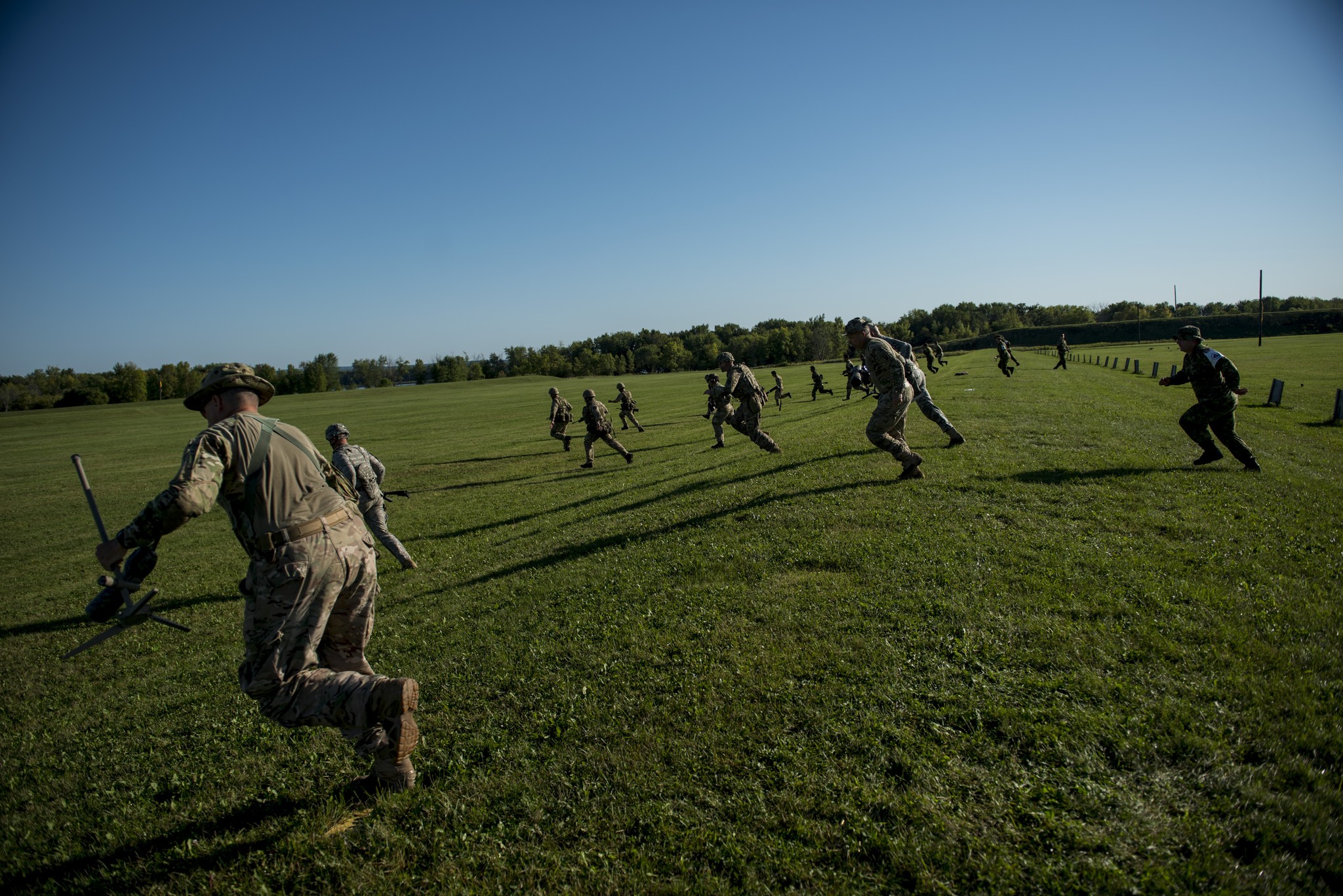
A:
<box><xmin>841</xmin><ymin>317</ymin><xmax>872</xmax><ymax>337</ymax></box>
<box><xmin>1172</xmin><ymin>325</ymin><xmax>1205</xmax><ymax>343</ymax></box>
<box><xmin>182</xmin><ymin>362</ymin><xmax>276</xmax><ymax>411</ymax></box>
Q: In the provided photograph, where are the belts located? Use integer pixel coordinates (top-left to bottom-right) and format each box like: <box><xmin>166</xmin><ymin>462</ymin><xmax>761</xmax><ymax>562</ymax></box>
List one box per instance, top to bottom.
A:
<box><xmin>905</xmin><ymin>365</ymin><xmax>919</xmax><ymax>371</ymax></box>
<box><xmin>256</xmin><ymin>504</ymin><xmax>355</xmax><ymax>553</ymax></box>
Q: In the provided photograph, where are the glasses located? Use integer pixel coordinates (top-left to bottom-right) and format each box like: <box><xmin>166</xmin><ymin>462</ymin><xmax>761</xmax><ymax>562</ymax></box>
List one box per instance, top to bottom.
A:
<box><xmin>717</xmin><ymin>361</ymin><xmax>721</xmax><ymax>365</ymax></box>
<box><xmin>199</xmin><ymin>397</ymin><xmax>213</xmax><ymax>419</ymax></box>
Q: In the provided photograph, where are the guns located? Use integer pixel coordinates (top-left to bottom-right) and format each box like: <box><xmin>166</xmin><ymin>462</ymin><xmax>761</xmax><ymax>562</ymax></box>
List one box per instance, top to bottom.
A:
<box><xmin>916</xmin><ymin>356</ymin><xmax>922</xmax><ymax>361</ymax></box>
<box><xmin>840</xmin><ymin>374</ymin><xmax>848</xmax><ymax>377</ymax></box>
<box><xmin>806</xmin><ymin>382</ymin><xmax>828</xmax><ymax>385</ymax></box>
<box><xmin>766</xmin><ymin>385</ymin><xmax>779</xmax><ymax>395</ymax></box>
<box><xmin>616</xmin><ymin>408</ymin><xmax>639</xmax><ymax>415</ymax></box>
<box><xmin>546</xmin><ymin>416</ymin><xmax>550</xmax><ymax>420</ymax></box>
<box><xmin>572</xmin><ymin>417</ymin><xmax>584</xmax><ymax>424</ymax></box>
<box><xmin>381</xmin><ymin>490</ymin><xmax>411</xmax><ymax>502</ymax></box>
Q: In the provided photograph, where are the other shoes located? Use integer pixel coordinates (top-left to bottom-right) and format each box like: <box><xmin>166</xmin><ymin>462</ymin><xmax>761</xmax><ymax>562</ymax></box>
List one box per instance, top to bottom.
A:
<box><xmin>932</xmin><ymin>361</ymin><xmax>948</xmax><ymax>375</ymax></box>
<box><xmin>702</xmin><ymin>414</ymin><xmax>710</xmax><ymax>420</ymax></box>
<box><xmin>401</xmin><ymin>558</ymin><xmax>418</xmax><ymax>571</ymax></box>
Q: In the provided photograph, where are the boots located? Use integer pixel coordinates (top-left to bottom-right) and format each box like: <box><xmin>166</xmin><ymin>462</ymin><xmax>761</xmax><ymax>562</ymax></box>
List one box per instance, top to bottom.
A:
<box><xmin>1006</xmin><ymin>361</ymin><xmax>1020</xmax><ymax>378</ymax></box>
<box><xmin>775</xmin><ymin>386</ymin><xmax>870</xmax><ymax>406</ymax></box>
<box><xmin>563</xmin><ymin>425</ymin><xmax>645</xmax><ymax>468</ymax></box>
<box><xmin>942</xmin><ymin>427</ymin><xmax>966</xmax><ymax>449</ymax></box>
<box><xmin>711</xmin><ymin>431</ymin><xmax>784</xmax><ymax>455</ymax></box>
<box><xmin>1193</xmin><ymin>440</ymin><xmax>1224</xmax><ymax>465</ymax></box>
<box><xmin>897</xmin><ymin>446</ymin><xmax>926</xmax><ymax>481</ymax></box>
<box><xmin>1236</xmin><ymin>456</ymin><xmax>1261</xmax><ymax>473</ymax></box>
<box><xmin>351</xmin><ymin>676</ymin><xmax>420</xmax><ymax>797</ymax></box>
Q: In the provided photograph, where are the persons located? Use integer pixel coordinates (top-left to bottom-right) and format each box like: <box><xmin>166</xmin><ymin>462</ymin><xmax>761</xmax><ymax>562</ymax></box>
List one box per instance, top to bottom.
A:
<box><xmin>841</xmin><ymin>318</ymin><xmax>926</xmax><ymax>481</ymax></box>
<box><xmin>867</xmin><ymin>322</ymin><xmax>966</xmax><ymax>451</ymax></box>
<box><xmin>1158</xmin><ymin>323</ymin><xmax>1262</xmax><ymax>475</ymax></box>
<box><xmin>1053</xmin><ymin>333</ymin><xmax>1070</xmax><ymax>371</ymax></box>
<box><xmin>994</xmin><ymin>335</ymin><xmax>1015</xmax><ymax>378</ymax></box>
<box><xmin>1001</xmin><ymin>336</ymin><xmax>1020</xmax><ymax>366</ymax></box>
<box><xmin>934</xmin><ymin>341</ymin><xmax>948</xmax><ymax>367</ymax></box>
<box><xmin>923</xmin><ymin>342</ymin><xmax>939</xmax><ymax>374</ymax></box>
<box><xmin>703</xmin><ymin>351</ymin><xmax>784</xmax><ymax>455</ymax></box>
<box><xmin>910</xmin><ymin>352</ymin><xmax>927</xmax><ymax>377</ymax></box>
<box><xmin>841</xmin><ymin>353</ymin><xmax>870</xmax><ymax>401</ymax></box>
<box><xmin>860</xmin><ymin>354</ymin><xmax>874</xmax><ymax>388</ymax></box>
<box><xmin>95</xmin><ymin>360</ymin><xmax>421</xmax><ymax>796</ymax></box>
<box><xmin>810</xmin><ymin>365</ymin><xmax>833</xmax><ymax>402</ymax></box>
<box><xmin>771</xmin><ymin>371</ymin><xmax>791</xmax><ymax>406</ymax></box>
<box><xmin>706</xmin><ymin>373</ymin><xmax>770</xmax><ymax>450</ymax></box>
<box><xmin>702</xmin><ymin>374</ymin><xmax>717</xmax><ymax>420</ymax></box>
<box><xmin>607</xmin><ymin>382</ymin><xmax>645</xmax><ymax>433</ymax></box>
<box><xmin>548</xmin><ymin>387</ymin><xmax>574</xmax><ymax>452</ymax></box>
<box><xmin>580</xmin><ymin>389</ymin><xmax>634</xmax><ymax>469</ymax></box>
<box><xmin>324</xmin><ymin>423</ymin><xmax>418</xmax><ymax>571</ymax></box>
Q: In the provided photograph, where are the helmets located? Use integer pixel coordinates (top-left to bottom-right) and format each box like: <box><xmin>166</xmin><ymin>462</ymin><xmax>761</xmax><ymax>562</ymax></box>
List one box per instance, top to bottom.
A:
<box><xmin>716</xmin><ymin>352</ymin><xmax>734</xmax><ymax>364</ymax></box>
<box><xmin>810</xmin><ymin>365</ymin><xmax>816</xmax><ymax>369</ymax></box>
<box><xmin>616</xmin><ymin>382</ymin><xmax>625</xmax><ymax>389</ymax></box>
<box><xmin>549</xmin><ymin>387</ymin><xmax>559</xmax><ymax>394</ymax></box>
<box><xmin>583</xmin><ymin>389</ymin><xmax>596</xmax><ymax>397</ymax></box>
<box><xmin>325</xmin><ymin>423</ymin><xmax>350</xmax><ymax>441</ymax></box>
